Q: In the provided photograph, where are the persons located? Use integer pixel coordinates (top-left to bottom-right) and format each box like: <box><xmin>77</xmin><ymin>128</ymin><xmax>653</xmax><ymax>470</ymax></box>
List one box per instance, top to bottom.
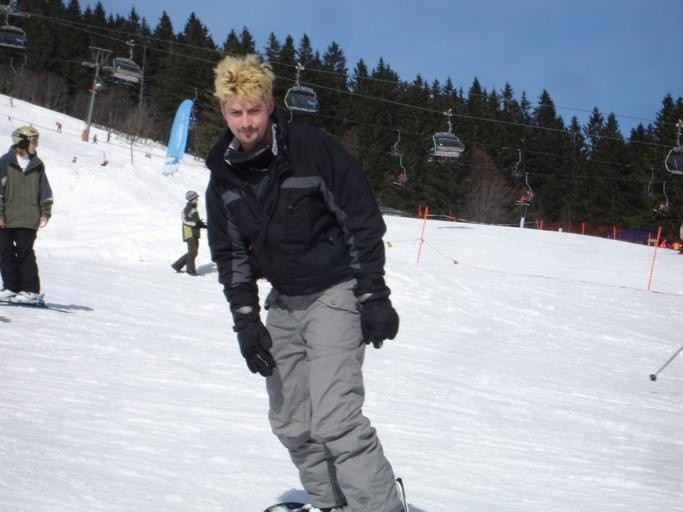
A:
<box><xmin>202</xmin><ymin>52</ymin><xmax>408</xmax><ymax>512</ymax></box>
<box><xmin>0</xmin><ymin>126</ymin><xmax>52</xmax><ymax>307</ymax></box>
<box><xmin>168</xmin><ymin>190</ymin><xmax>207</xmax><ymax>276</ymax></box>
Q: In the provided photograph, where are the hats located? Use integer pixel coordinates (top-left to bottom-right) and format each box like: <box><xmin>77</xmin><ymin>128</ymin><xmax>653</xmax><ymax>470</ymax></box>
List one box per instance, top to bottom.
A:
<box><xmin>185</xmin><ymin>190</ymin><xmax>200</xmax><ymax>201</ymax></box>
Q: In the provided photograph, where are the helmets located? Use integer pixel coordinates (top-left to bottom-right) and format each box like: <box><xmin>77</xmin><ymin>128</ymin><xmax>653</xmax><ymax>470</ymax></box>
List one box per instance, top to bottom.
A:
<box><xmin>12</xmin><ymin>126</ymin><xmax>39</xmax><ymax>145</ymax></box>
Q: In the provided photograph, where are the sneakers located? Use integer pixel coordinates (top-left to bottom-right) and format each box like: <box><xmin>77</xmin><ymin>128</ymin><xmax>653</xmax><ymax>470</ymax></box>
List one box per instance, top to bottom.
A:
<box><xmin>172</xmin><ymin>264</ymin><xmax>184</xmax><ymax>273</ymax></box>
<box><xmin>188</xmin><ymin>272</ymin><xmax>196</xmax><ymax>276</ymax></box>
<box><xmin>0</xmin><ymin>289</ymin><xmax>39</xmax><ymax>304</ymax></box>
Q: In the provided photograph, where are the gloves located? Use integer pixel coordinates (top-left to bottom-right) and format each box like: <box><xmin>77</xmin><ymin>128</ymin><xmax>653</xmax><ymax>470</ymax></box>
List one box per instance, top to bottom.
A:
<box><xmin>196</xmin><ymin>220</ymin><xmax>207</xmax><ymax>228</ymax></box>
<box><xmin>352</xmin><ymin>282</ymin><xmax>399</xmax><ymax>349</ymax></box>
<box><xmin>232</xmin><ymin>304</ymin><xmax>276</xmax><ymax>377</ymax></box>
